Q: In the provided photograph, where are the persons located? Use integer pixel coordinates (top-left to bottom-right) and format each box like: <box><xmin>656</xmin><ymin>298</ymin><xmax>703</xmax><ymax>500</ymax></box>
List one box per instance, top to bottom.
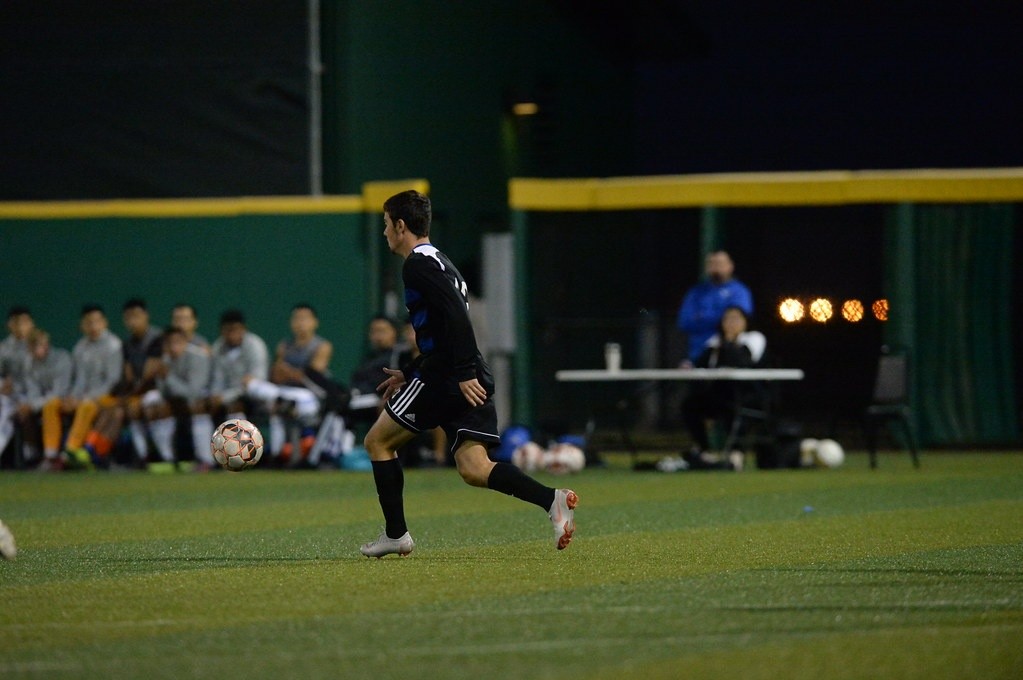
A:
<box><xmin>360</xmin><ymin>187</ymin><xmax>579</xmax><ymax>562</ymax></box>
<box><xmin>678</xmin><ymin>247</ymin><xmax>770</xmax><ymax>466</ymax></box>
<box><xmin>1</xmin><ymin>297</ymin><xmax>459</xmax><ymax>470</ymax></box>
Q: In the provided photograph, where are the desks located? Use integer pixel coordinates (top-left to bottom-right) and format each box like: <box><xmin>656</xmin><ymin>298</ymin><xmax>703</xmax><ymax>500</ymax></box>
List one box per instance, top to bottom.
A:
<box><xmin>556</xmin><ymin>368</ymin><xmax>805</xmax><ymax>464</ymax></box>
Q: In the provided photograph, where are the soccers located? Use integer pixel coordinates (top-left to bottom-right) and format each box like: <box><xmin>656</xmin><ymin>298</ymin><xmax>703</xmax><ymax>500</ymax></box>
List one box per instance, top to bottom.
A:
<box><xmin>210</xmin><ymin>420</ymin><xmax>266</xmax><ymax>471</ymax></box>
<box><xmin>802</xmin><ymin>439</ymin><xmax>845</xmax><ymax>468</ymax></box>
<box><xmin>512</xmin><ymin>442</ymin><xmax>585</xmax><ymax>474</ymax></box>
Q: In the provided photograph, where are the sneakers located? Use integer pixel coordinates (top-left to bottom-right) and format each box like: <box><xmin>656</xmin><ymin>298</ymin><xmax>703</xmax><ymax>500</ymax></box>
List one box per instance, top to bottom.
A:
<box><xmin>547</xmin><ymin>489</ymin><xmax>579</xmax><ymax>549</ymax></box>
<box><xmin>359</xmin><ymin>526</ymin><xmax>413</xmax><ymax>559</ymax></box>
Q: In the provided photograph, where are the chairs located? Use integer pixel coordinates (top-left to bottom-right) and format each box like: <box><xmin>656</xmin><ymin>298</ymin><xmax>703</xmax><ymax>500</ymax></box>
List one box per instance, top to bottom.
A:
<box><xmin>825</xmin><ymin>342</ymin><xmax>920</xmax><ymax>475</ymax></box>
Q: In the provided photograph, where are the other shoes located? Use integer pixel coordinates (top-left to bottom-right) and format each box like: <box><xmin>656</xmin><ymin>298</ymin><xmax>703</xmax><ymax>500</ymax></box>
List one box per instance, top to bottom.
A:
<box><xmin>61</xmin><ymin>447</ymin><xmax>90</xmax><ymax>467</ymax></box>
<box><xmin>681</xmin><ymin>448</ymin><xmax>714</xmax><ymax>468</ymax></box>
<box><xmin>726</xmin><ymin>451</ymin><xmax>745</xmax><ymax>471</ymax></box>
<box><xmin>38</xmin><ymin>456</ymin><xmax>63</xmax><ymax>471</ymax></box>
<box><xmin>147</xmin><ymin>459</ymin><xmax>175</xmax><ymax>474</ymax></box>
<box><xmin>178</xmin><ymin>459</ymin><xmax>197</xmax><ymax>471</ymax></box>
<box><xmin>0</xmin><ymin>519</ymin><xmax>17</xmax><ymax>560</ymax></box>
